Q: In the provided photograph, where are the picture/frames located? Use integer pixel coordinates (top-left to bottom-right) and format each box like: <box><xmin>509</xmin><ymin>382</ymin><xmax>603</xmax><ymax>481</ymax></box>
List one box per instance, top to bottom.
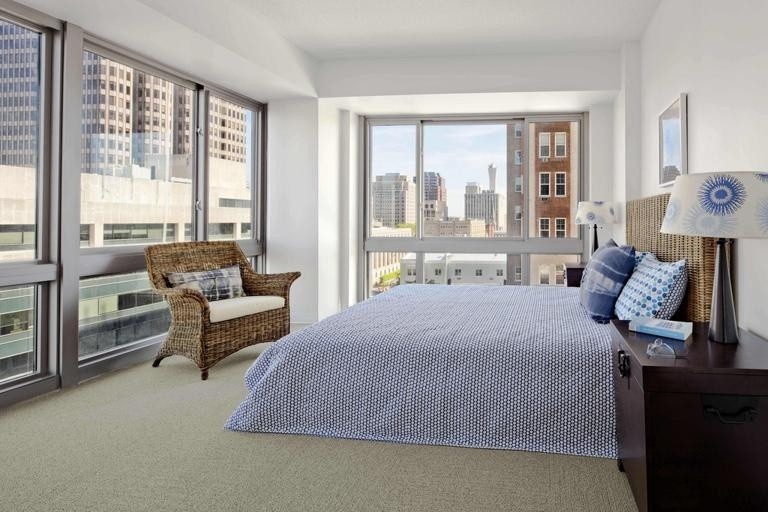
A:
<box><xmin>657</xmin><ymin>92</ymin><xmax>689</xmax><ymax>187</ymax></box>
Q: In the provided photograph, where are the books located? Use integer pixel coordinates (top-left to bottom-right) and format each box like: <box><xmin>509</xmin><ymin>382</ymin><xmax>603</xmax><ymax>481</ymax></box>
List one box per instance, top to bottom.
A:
<box><xmin>628</xmin><ymin>315</ymin><xmax>695</xmax><ymax>342</ymax></box>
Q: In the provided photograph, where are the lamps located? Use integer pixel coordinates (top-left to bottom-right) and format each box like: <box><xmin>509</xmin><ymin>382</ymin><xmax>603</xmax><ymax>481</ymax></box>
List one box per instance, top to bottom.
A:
<box><xmin>657</xmin><ymin>167</ymin><xmax>768</xmax><ymax>348</ymax></box>
<box><xmin>573</xmin><ymin>199</ymin><xmax>620</xmax><ymax>254</ymax></box>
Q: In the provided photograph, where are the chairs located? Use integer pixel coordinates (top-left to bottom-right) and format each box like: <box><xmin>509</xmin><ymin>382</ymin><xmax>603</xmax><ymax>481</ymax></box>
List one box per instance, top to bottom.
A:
<box><xmin>143</xmin><ymin>239</ymin><xmax>302</xmax><ymax>381</ymax></box>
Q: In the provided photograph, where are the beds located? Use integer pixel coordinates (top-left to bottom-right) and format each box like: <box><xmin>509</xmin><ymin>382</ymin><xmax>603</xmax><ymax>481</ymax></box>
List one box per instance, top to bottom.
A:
<box><xmin>228</xmin><ymin>195</ymin><xmax>726</xmax><ymax>461</ymax></box>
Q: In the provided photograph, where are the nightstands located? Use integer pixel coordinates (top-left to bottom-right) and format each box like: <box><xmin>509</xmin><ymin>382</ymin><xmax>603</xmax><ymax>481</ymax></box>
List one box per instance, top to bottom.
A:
<box><xmin>561</xmin><ymin>261</ymin><xmax>586</xmax><ymax>285</ymax></box>
<box><xmin>609</xmin><ymin>318</ymin><xmax>768</xmax><ymax>512</ymax></box>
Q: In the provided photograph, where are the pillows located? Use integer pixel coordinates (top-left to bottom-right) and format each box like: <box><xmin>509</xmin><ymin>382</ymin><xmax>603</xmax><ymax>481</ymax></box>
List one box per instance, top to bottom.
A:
<box><xmin>576</xmin><ymin>236</ymin><xmax>688</xmax><ymax>327</ymax></box>
<box><xmin>162</xmin><ymin>262</ymin><xmax>248</xmax><ymax>303</ymax></box>
